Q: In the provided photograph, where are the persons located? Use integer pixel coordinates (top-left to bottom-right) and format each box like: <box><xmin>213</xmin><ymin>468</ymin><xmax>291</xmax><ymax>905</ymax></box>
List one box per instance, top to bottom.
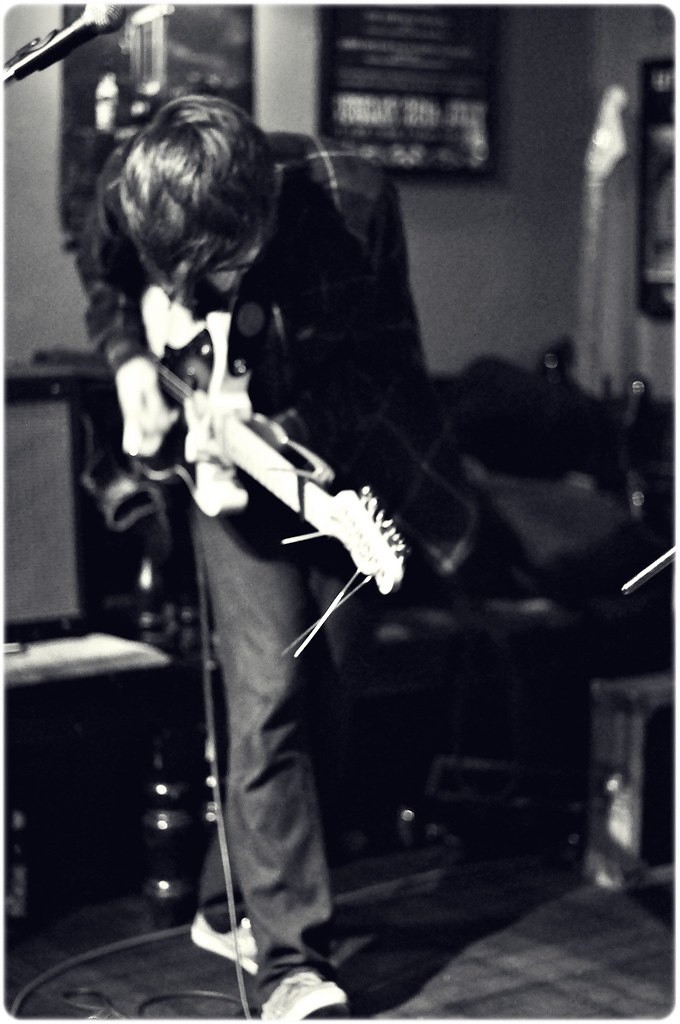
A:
<box><xmin>77</xmin><ymin>95</ymin><xmax>439</xmax><ymax>1019</ymax></box>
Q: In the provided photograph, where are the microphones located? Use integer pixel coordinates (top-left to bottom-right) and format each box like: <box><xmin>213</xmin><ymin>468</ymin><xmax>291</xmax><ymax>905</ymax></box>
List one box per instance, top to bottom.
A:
<box><xmin>8</xmin><ymin>4</ymin><xmax>128</xmax><ymax>84</ymax></box>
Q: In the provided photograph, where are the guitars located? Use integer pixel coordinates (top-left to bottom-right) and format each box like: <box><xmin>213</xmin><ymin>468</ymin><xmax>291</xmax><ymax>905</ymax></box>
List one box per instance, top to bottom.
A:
<box><xmin>132</xmin><ymin>284</ymin><xmax>413</xmax><ymax>598</ymax></box>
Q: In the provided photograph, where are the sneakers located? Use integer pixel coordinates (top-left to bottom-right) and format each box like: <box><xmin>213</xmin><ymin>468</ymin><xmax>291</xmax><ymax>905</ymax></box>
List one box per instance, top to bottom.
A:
<box><xmin>191</xmin><ymin>910</ymin><xmax>258</xmax><ymax>975</ymax></box>
<box><xmin>260</xmin><ymin>971</ymin><xmax>348</xmax><ymax>1020</ymax></box>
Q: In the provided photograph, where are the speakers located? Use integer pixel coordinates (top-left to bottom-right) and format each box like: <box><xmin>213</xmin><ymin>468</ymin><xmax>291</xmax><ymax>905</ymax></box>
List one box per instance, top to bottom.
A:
<box><xmin>4</xmin><ymin>365</ymin><xmax>103</xmax><ymax>644</ymax></box>
